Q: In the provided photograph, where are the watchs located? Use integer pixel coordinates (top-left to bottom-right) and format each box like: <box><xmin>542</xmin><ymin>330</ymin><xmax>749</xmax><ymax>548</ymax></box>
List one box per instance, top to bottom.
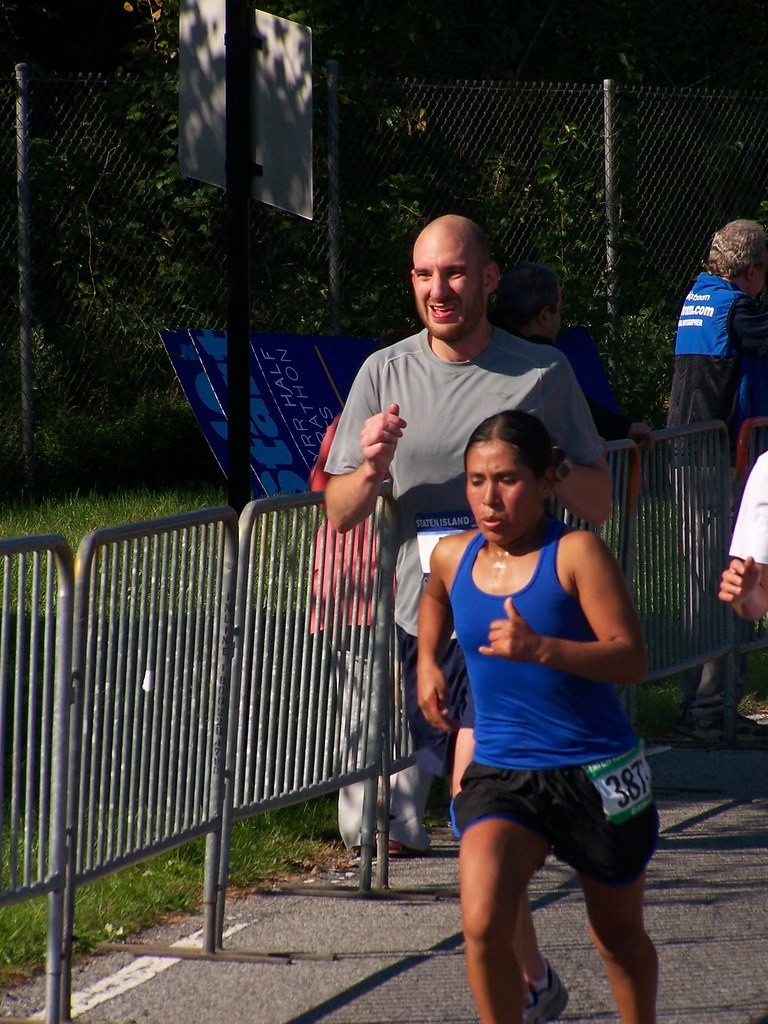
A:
<box><xmin>547</xmin><ymin>456</ymin><xmax>574</xmax><ymax>488</ymax></box>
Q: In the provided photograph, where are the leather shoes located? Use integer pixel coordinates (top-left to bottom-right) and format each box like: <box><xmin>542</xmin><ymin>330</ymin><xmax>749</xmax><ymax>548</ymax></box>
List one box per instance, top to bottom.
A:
<box><xmin>386</xmin><ymin>839</ymin><xmax>435</xmax><ymax>857</ymax></box>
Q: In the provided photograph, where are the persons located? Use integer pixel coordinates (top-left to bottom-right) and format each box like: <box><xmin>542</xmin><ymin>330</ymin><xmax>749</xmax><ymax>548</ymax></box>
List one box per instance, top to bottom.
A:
<box><xmin>321</xmin><ymin>213</ymin><xmax>612</xmax><ymax>1024</ymax></box>
<box><xmin>666</xmin><ymin>219</ymin><xmax>767</xmax><ymax>752</ymax></box>
<box><xmin>490</xmin><ymin>262</ymin><xmax>655</xmax><ymax>448</ymax></box>
<box><xmin>309</xmin><ymin>408</ymin><xmax>430</xmax><ymax>872</ymax></box>
<box><xmin>415</xmin><ymin>408</ymin><xmax>666</xmax><ymax>1024</ymax></box>
<box><xmin>718</xmin><ymin>446</ymin><xmax>768</xmax><ymax>623</ymax></box>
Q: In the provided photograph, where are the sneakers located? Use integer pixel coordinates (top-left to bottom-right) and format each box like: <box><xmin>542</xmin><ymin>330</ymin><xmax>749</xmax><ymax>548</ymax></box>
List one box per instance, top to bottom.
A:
<box><xmin>516</xmin><ymin>962</ymin><xmax>567</xmax><ymax>1022</ymax></box>
